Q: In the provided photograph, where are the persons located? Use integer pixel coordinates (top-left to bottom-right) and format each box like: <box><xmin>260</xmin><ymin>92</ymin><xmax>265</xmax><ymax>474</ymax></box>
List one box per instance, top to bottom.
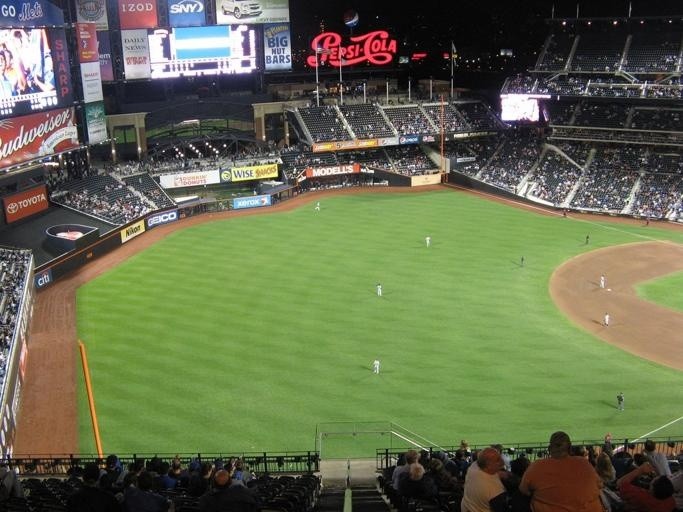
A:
<box><xmin>1</xmin><ymin>28</ymin><xmax>57</xmax><ymax>106</ymax></box>
<box><xmin>586</xmin><ymin>235</ymin><xmax>589</xmax><ymax>244</ymax></box>
<box><xmin>425</xmin><ymin>236</ymin><xmax>431</xmax><ymax>248</ymax></box>
<box><xmin>0</xmin><ymin>247</ymin><xmax>31</xmax><ymax>434</ymax></box>
<box><xmin>521</xmin><ymin>256</ymin><xmax>524</xmax><ymax>265</ymax></box>
<box><xmin>600</xmin><ymin>273</ymin><xmax>606</xmax><ymax>288</ymax></box>
<box><xmin>376</xmin><ymin>284</ymin><xmax>382</xmax><ymax>297</ymax></box>
<box><xmin>602</xmin><ymin>312</ymin><xmax>609</xmax><ymax>326</ymax></box>
<box><xmin>373</xmin><ymin>358</ymin><xmax>380</xmax><ymax>374</ymax></box>
<box><xmin>617</xmin><ymin>392</ymin><xmax>624</xmax><ymax>412</ymax></box>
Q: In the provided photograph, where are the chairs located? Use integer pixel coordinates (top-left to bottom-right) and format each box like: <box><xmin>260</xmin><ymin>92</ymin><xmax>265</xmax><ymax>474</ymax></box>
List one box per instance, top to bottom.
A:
<box><xmin>0</xmin><ymin>473</ymin><xmax>317</xmax><ymax>512</ymax></box>
